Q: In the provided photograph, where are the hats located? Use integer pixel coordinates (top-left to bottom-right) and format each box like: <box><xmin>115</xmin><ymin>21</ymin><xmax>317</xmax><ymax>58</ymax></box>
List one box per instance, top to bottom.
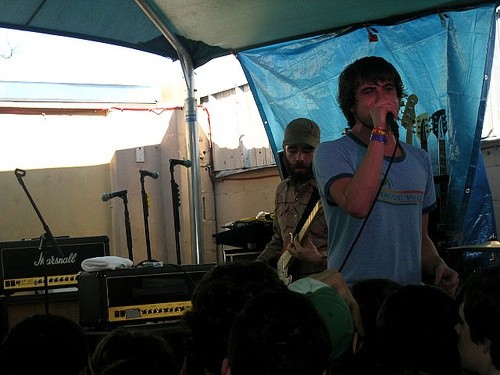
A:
<box><xmin>284</xmin><ymin>118</ymin><xmax>320</xmax><ymax>149</ymax></box>
<box><xmin>287</xmin><ymin>277</ymin><xmax>354</xmax><ymax>361</ymax></box>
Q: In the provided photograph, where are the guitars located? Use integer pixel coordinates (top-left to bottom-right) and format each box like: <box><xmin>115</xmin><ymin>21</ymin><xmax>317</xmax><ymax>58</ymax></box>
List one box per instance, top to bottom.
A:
<box><xmin>397</xmin><ymin>95</ymin><xmax>451</xmax><ymax>247</ymax></box>
<box><xmin>277</xmin><ymin>199</ymin><xmax>323</xmax><ymax>285</ymax></box>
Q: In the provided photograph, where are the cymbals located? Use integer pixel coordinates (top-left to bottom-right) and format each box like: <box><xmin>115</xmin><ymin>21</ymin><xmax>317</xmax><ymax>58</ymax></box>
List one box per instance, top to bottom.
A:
<box><xmin>446</xmin><ymin>241</ymin><xmax>500</xmax><ymax>252</ymax></box>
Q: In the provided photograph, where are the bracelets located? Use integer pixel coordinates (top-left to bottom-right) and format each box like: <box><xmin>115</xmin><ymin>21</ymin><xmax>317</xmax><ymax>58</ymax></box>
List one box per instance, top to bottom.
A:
<box><xmin>369</xmin><ymin>127</ymin><xmax>388</xmax><ymax>144</ymax></box>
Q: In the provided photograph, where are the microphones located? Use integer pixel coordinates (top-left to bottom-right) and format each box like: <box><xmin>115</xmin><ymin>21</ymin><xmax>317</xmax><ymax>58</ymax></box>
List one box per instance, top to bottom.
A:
<box><xmin>386</xmin><ymin>112</ymin><xmax>399</xmax><ymax>138</ymax></box>
<box><xmin>169</xmin><ymin>159</ymin><xmax>192</xmax><ymax>167</ymax></box>
<box><xmin>139</xmin><ymin>169</ymin><xmax>159</xmax><ymax>179</ymax></box>
<box><xmin>101</xmin><ymin>189</ymin><xmax>127</xmax><ymax>201</ymax></box>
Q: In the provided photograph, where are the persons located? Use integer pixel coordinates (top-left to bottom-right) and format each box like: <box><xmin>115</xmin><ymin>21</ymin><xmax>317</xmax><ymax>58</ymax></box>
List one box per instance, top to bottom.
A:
<box><xmin>312</xmin><ymin>56</ymin><xmax>460</xmax><ymax>295</ymax></box>
<box><xmin>254</xmin><ymin>118</ymin><xmax>329</xmax><ymax>284</ymax></box>
<box><xmin>0</xmin><ymin>262</ymin><xmax>500</xmax><ymax>375</ymax></box>
